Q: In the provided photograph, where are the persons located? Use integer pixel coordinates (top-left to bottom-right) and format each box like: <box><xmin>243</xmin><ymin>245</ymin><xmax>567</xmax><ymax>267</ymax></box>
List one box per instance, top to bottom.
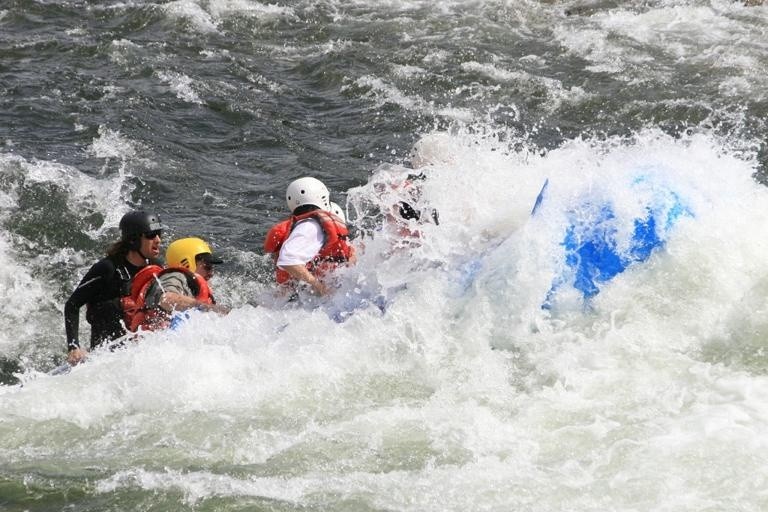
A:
<box><xmin>384</xmin><ymin>131</ymin><xmax>457</xmax><ymax>240</ymax></box>
<box><xmin>263</xmin><ymin>176</ymin><xmax>355</xmax><ymax>298</ymax></box>
<box><xmin>65</xmin><ymin>210</ymin><xmax>170</xmax><ymax>369</ymax></box>
<box><xmin>121</xmin><ymin>237</ymin><xmax>231</xmax><ymax>341</ymax></box>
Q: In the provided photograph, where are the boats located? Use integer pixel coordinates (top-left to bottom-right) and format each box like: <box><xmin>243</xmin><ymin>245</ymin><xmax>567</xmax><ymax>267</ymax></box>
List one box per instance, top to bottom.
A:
<box><xmin>168</xmin><ymin>169</ymin><xmax>694</xmax><ymax>333</ymax></box>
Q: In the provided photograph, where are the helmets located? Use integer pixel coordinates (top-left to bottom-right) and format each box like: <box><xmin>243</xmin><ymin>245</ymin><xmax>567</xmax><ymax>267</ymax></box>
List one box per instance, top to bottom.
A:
<box><xmin>286</xmin><ymin>175</ymin><xmax>344</xmax><ymax>221</ymax></box>
<box><xmin>119</xmin><ymin>209</ymin><xmax>165</xmax><ymax>240</ymax></box>
<box><xmin>165</xmin><ymin>236</ymin><xmax>225</xmax><ymax>273</ymax></box>
